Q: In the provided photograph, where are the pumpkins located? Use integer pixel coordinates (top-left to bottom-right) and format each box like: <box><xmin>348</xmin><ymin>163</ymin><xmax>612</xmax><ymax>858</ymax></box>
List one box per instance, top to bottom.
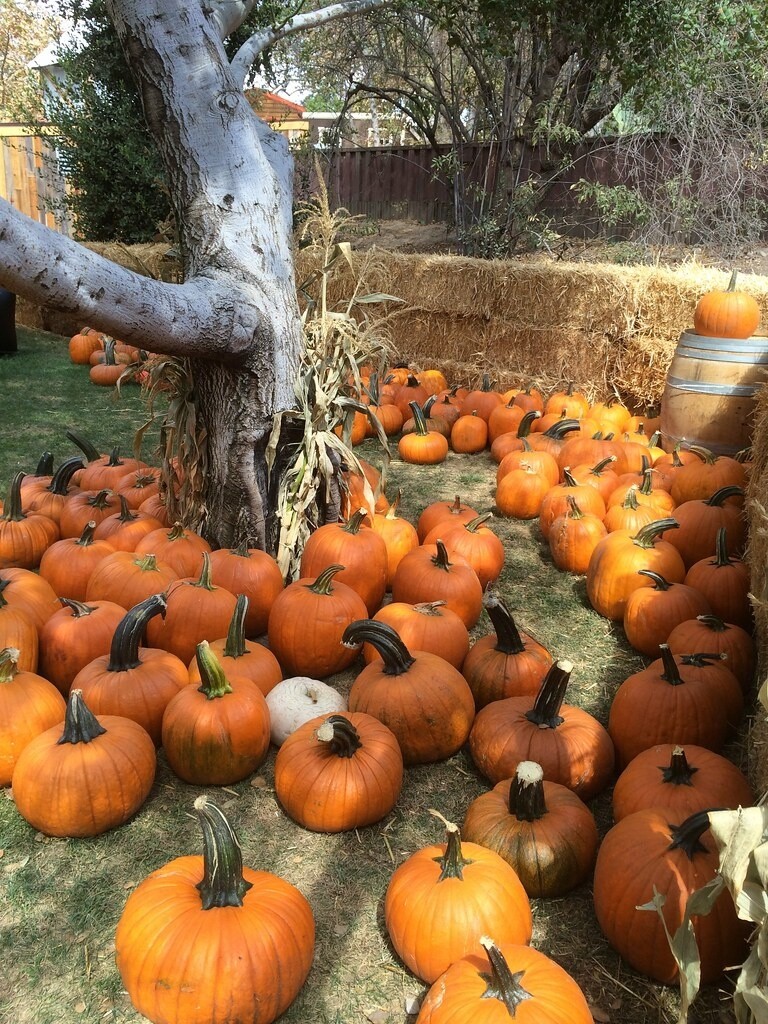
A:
<box><xmin>693</xmin><ymin>268</ymin><xmax>760</xmax><ymax>338</ymax></box>
<box><xmin>0</xmin><ymin>327</ymin><xmax>754</xmax><ymax>1024</ymax></box>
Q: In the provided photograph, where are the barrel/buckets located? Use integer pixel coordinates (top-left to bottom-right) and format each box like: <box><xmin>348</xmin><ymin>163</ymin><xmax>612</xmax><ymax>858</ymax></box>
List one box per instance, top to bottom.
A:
<box><xmin>660</xmin><ymin>328</ymin><xmax>768</xmax><ymax>458</ymax></box>
